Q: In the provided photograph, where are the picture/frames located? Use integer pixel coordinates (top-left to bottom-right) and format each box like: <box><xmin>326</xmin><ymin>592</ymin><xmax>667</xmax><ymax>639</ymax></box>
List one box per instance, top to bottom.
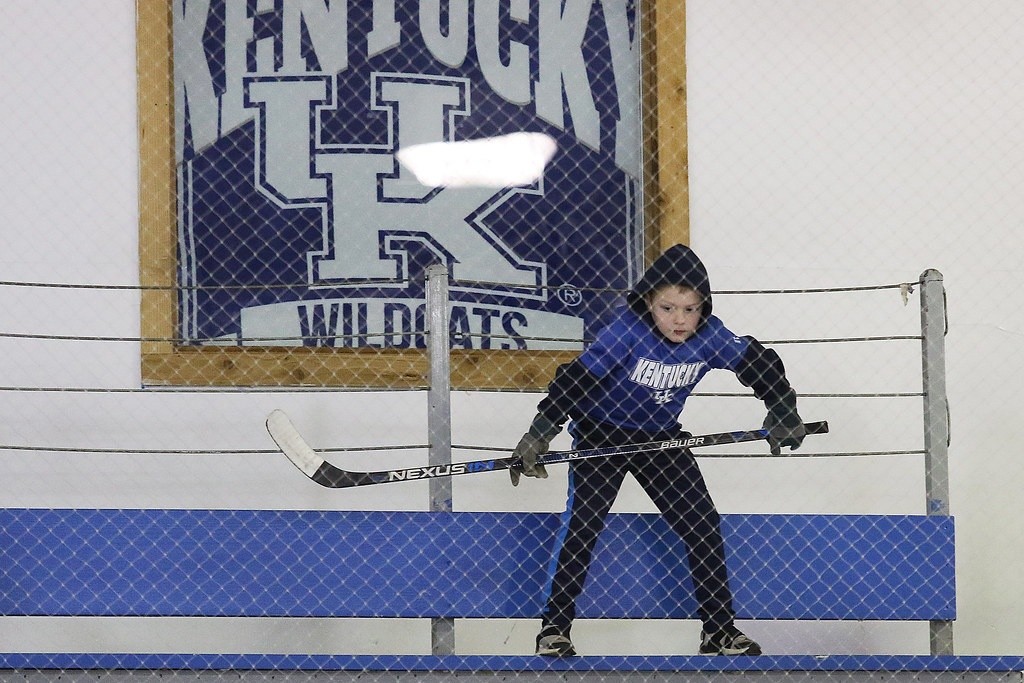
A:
<box><xmin>135</xmin><ymin>0</ymin><xmax>692</xmax><ymax>388</ymax></box>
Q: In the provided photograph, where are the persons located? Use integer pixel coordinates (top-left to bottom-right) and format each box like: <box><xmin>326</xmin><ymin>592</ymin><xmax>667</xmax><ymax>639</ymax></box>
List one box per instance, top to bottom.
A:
<box><xmin>509</xmin><ymin>243</ymin><xmax>805</xmax><ymax>655</ymax></box>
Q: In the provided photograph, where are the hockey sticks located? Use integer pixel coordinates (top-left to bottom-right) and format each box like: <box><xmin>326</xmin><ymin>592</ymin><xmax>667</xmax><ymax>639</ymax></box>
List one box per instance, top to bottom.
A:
<box><xmin>263</xmin><ymin>404</ymin><xmax>835</xmax><ymax>491</ymax></box>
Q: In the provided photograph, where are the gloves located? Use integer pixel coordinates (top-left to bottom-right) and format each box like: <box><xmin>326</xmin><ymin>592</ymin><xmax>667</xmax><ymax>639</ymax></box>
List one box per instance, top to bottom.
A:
<box><xmin>764</xmin><ymin>389</ymin><xmax>806</xmax><ymax>456</ymax></box>
<box><xmin>510</xmin><ymin>414</ymin><xmax>563</xmax><ymax>486</ymax></box>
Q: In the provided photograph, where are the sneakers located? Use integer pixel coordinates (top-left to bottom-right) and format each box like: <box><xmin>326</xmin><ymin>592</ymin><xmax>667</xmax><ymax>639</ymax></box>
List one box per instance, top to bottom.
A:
<box><xmin>698</xmin><ymin>623</ymin><xmax>762</xmax><ymax>656</ymax></box>
<box><xmin>534</xmin><ymin>632</ymin><xmax>575</xmax><ymax>656</ymax></box>
